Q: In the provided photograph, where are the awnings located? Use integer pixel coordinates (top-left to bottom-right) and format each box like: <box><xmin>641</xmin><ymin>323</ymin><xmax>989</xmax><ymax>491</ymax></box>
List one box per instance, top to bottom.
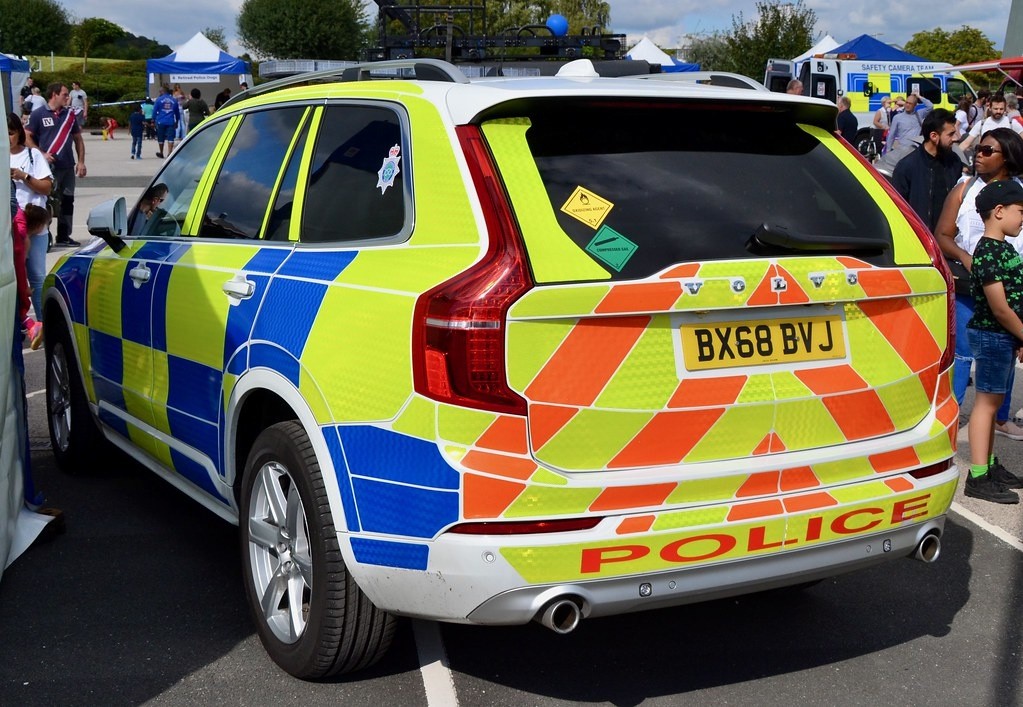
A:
<box><xmin>920</xmin><ymin>57</ymin><xmax>1023</xmax><ymax>103</ymax></box>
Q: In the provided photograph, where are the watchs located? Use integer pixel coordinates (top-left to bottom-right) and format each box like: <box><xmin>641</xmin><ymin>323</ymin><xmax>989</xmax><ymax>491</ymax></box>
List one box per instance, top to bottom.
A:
<box><xmin>23</xmin><ymin>174</ymin><xmax>31</xmax><ymax>184</ymax></box>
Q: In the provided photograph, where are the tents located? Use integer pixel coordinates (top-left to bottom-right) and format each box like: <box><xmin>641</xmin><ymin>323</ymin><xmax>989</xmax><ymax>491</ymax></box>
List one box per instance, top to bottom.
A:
<box><xmin>146</xmin><ymin>31</ymin><xmax>254</xmax><ymax>97</ymax></box>
<box><xmin>801</xmin><ymin>34</ymin><xmax>930</xmax><ymax>61</ymax></box>
<box><xmin>789</xmin><ymin>35</ymin><xmax>843</xmax><ymax>62</ymax></box>
<box><xmin>621</xmin><ymin>37</ymin><xmax>699</xmax><ymax>72</ymax></box>
<box><xmin>0</xmin><ymin>52</ymin><xmax>31</xmax><ymax>118</ymax></box>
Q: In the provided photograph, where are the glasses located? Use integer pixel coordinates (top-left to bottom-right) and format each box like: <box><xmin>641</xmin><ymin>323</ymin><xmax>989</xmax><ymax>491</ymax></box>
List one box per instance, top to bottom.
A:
<box><xmin>975</xmin><ymin>145</ymin><xmax>1001</xmax><ymax>157</ymax></box>
<box><xmin>158</xmin><ymin>199</ymin><xmax>164</xmax><ymax>202</ymax></box>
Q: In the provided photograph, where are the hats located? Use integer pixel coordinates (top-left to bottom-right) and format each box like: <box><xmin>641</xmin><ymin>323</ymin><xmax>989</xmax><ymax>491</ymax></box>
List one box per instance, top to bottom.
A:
<box><xmin>975</xmin><ymin>180</ymin><xmax>1023</xmax><ymax>212</ymax></box>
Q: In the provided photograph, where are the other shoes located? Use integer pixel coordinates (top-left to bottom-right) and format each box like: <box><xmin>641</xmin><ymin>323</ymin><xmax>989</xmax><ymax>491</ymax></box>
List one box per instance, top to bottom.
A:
<box><xmin>131</xmin><ymin>155</ymin><xmax>134</xmax><ymax>159</ymax></box>
<box><xmin>136</xmin><ymin>156</ymin><xmax>142</xmax><ymax>159</ymax></box>
<box><xmin>994</xmin><ymin>420</ymin><xmax>1023</xmax><ymax>440</ymax></box>
<box><xmin>987</xmin><ymin>456</ymin><xmax>1022</xmax><ymax>489</ymax></box>
<box><xmin>963</xmin><ymin>468</ymin><xmax>1019</xmax><ymax>503</ymax></box>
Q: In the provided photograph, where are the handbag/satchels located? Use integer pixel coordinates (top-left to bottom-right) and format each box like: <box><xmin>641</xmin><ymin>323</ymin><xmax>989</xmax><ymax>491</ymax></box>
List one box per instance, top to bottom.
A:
<box><xmin>23</xmin><ymin>102</ymin><xmax>33</xmax><ymax>114</ymax></box>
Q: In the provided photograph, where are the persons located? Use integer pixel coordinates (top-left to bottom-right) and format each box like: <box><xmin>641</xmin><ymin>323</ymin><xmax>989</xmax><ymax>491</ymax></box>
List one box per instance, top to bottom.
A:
<box><xmin>7</xmin><ymin>77</ymin><xmax>88</xmax><ymax>350</ymax></box>
<box><xmin>787</xmin><ymin>80</ymin><xmax>803</xmax><ymax>95</ymax></box>
<box><xmin>239</xmin><ymin>82</ymin><xmax>249</xmax><ymax>91</ymax></box>
<box><xmin>128</xmin><ymin>84</ymin><xmax>185</xmax><ymax>159</ymax></box>
<box><xmin>182</xmin><ymin>88</ymin><xmax>210</xmax><ymax>130</ymax></box>
<box><xmin>215</xmin><ymin>88</ymin><xmax>231</xmax><ymax>110</ymax></box>
<box><xmin>873</xmin><ymin>89</ymin><xmax>1023</xmax><ymax>238</ymax></box>
<box><xmin>835</xmin><ymin>97</ymin><xmax>859</xmax><ymax>146</ymax></box>
<box><xmin>100</xmin><ymin>117</ymin><xmax>118</xmax><ymax>139</ymax></box>
<box><xmin>934</xmin><ymin>127</ymin><xmax>1022</xmax><ymax>440</ymax></box>
<box><xmin>964</xmin><ymin>180</ymin><xmax>1023</xmax><ymax>504</ymax></box>
<box><xmin>129</xmin><ymin>183</ymin><xmax>169</xmax><ymax>236</ymax></box>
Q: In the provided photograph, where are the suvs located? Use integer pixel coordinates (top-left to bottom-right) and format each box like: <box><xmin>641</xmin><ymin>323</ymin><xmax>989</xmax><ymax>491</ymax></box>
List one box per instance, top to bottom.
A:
<box><xmin>39</xmin><ymin>57</ymin><xmax>961</xmax><ymax>679</ymax></box>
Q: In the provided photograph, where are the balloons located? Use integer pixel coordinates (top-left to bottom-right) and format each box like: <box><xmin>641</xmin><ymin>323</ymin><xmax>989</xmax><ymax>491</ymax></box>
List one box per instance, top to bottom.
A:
<box><xmin>546</xmin><ymin>15</ymin><xmax>568</xmax><ymax>35</ymax></box>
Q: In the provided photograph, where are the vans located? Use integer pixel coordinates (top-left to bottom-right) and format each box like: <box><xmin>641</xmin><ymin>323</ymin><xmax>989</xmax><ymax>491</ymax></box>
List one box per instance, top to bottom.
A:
<box><xmin>764</xmin><ymin>54</ymin><xmax>980</xmax><ymax>159</ymax></box>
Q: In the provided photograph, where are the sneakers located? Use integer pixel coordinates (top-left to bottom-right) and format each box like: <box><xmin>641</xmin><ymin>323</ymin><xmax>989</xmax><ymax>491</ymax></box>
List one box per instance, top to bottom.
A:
<box><xmin>156</xmin><ymin>152</ymin><xmax>164</xmax><ymax>158</ymax></box>
<box><xmin>55</xmin><ymin>236</ymin><xmax>82</xmax><ymax>247</ymax></box>
<box><xmin>28</xmin><ymin>321</ymin><xmax>43</xmax><ymax>351</ymax></box>
